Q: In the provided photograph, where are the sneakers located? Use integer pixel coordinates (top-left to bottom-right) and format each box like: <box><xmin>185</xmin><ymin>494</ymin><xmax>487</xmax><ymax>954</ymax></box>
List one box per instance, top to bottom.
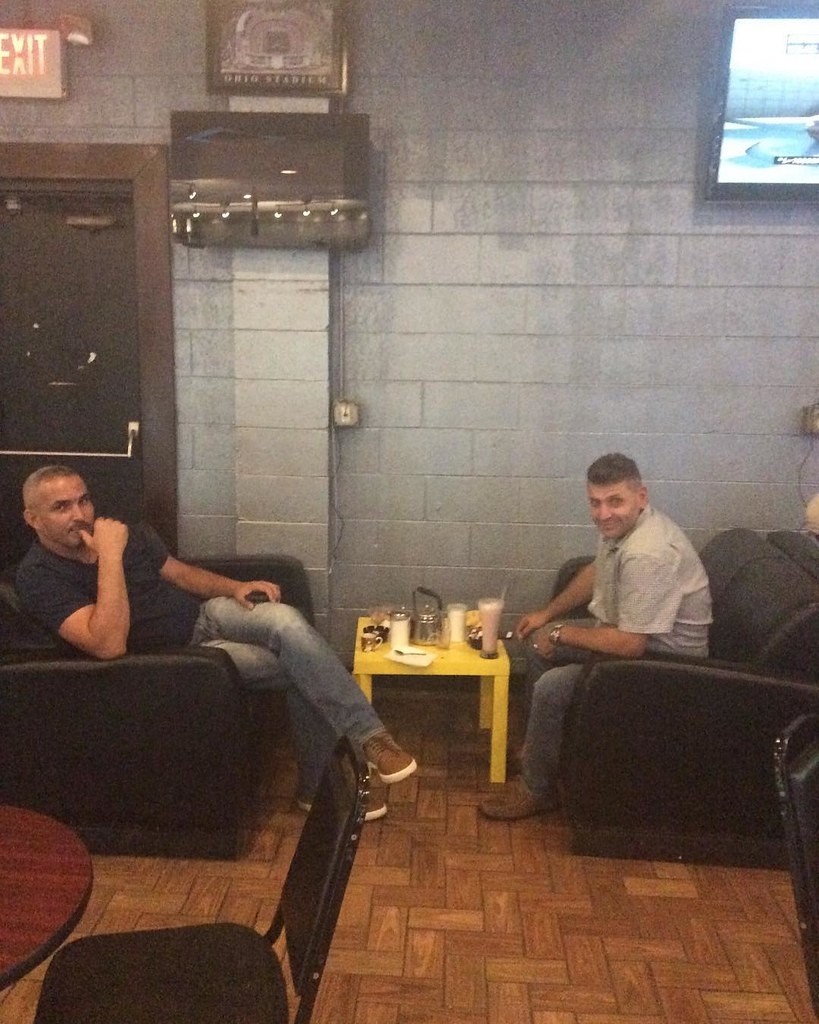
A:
<box><xmin>363</xmin><ymin>731</ymin><xmax>417</xmax><ymax>784</ymax></box>
<box><xmin>295</xmin><ymin>794</ymin><xmax>387</xmax><ymax>821</ymax></box>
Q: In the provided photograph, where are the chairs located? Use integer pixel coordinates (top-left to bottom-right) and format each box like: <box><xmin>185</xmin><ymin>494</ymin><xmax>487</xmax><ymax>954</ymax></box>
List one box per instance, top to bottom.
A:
<box><xmin>32</xmin><ymin>923</ymin><xmax>293</xmax><ymax>1024</ymax></box>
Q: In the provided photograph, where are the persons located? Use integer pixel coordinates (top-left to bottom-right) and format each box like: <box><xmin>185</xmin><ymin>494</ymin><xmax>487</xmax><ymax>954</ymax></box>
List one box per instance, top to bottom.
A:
<box><xmin>480</xmin><ymin>454</ymin><xmax>713</xmax><ymax>822</ymax></box>
<box><xmin>16</xmin><ymin>465</ymin><xmax>416</xmax><ymax>820</ymax></box>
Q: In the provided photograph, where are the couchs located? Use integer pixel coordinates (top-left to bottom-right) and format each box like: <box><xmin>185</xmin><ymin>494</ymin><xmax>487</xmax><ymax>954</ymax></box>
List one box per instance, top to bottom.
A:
<box><xmin>560</xmin><ymin>525</ymin><xmax>819</xmax><ymax>868</ymax></box>
<box><xmin>0</xmin><ymin>555</ymin><xmax>314</xmax><ymax>857</ymax></box>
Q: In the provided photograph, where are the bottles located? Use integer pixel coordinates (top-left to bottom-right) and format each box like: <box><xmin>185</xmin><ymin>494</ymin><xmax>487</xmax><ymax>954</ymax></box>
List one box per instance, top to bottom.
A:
<box><xmin>390</xmin><ymin>610</ymin><xmax>411</xmax><ymax>650</ymax></box>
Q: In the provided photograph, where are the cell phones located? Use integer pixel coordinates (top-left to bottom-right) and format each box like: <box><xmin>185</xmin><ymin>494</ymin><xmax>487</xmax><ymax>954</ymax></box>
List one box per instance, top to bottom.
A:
<box><xmin>245</xmin><ymin>591</ymin><xmax>271</xmax><ymax>603</ymax></box>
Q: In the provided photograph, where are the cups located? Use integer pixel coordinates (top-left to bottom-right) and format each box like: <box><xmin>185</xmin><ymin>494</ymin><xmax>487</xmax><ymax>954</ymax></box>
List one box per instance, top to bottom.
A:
<box><xmin>478</xmin><ymin>597</ymin><xmax>505</xmax><ymax>658</ymax></box>
<box><xmin>447</xmin><ymin>602</ymin><xmax>468</xmax><ymax>645</ymax></box>
<box><xmin>360</xmin><ymin>633</ymin><xmax>383</xmax><ymax>652</ymax></box>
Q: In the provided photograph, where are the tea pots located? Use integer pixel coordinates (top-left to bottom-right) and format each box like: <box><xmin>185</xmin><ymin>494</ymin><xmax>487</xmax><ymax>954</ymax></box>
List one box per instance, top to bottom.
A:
<box><xmin>400</xmin><ymin>586</ymin><xmax>443</xmax><ymax>646</ymax></box>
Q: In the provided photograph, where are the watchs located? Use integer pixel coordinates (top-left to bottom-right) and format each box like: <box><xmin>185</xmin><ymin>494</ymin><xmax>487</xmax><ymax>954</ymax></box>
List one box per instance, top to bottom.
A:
<box><xmin>549</xmin><ymin>624</ymin><xmax>566</xmax><ymax>646</ymax></box>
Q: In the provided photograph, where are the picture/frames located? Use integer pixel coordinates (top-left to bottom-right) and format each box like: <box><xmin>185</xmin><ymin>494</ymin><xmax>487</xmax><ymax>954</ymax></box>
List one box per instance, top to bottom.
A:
<box><xmin>207</xmin><ymin>0</ymin><xmax>347</xmax><ymax>98</ymax></box>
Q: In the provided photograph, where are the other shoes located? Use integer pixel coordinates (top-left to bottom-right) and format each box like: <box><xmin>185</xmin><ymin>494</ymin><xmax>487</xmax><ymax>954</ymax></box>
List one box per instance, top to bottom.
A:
<box><xmin>452</xmin><ymin>730</ymin><xmax>519</xmax><ymax>762</ymax></box>
<box><xmin>480</xmin><ymin>775</ymin><xmax>562</xmax><ymax>820</ymax></box>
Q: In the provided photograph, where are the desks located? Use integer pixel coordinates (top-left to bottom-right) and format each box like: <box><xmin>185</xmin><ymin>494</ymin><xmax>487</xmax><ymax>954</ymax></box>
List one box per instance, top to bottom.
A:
<box><xmin>354</xmin><ymin>607</ymin><xmax>511</xmax><ymax>784</ymax></box>
<box><xmin>0</xmin><ymin>802</ymin><xmax>93</xmax><ymax>995</ymax></box>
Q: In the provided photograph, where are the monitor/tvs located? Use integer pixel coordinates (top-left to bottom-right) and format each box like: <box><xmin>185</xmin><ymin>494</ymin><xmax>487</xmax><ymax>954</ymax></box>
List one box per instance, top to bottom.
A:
<box><xmin>704</xmin><ymin>0</ymin><xmax>819</xmax><ymax>206</ymax></box>
